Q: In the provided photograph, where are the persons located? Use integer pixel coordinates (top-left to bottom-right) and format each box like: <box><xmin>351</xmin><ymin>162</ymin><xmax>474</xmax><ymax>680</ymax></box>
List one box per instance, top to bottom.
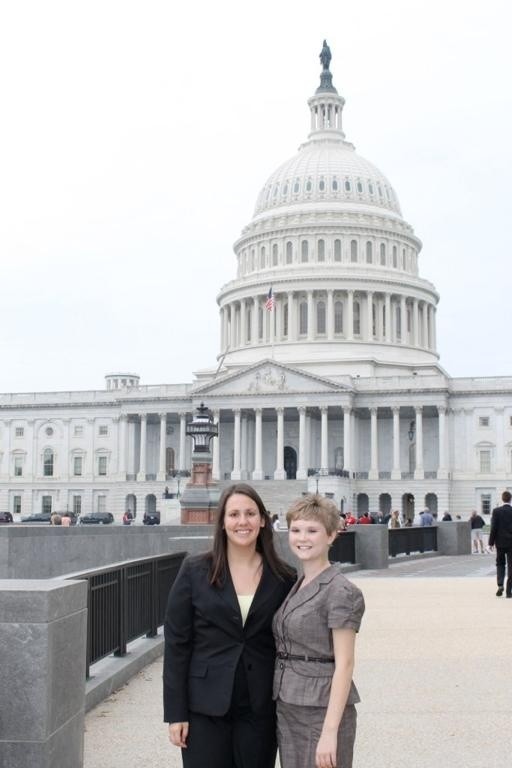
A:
<box><xmin>318</xmin><ymin>39</ymin><xmax>333</xmax><ymax>71</ymax></box>
<box><xmin>162</xmin><ymin>485</ymin><xmax>287</xmax><ymax>766</ymax></box>
<box><xmin>489</xmin><ymin>491</ymin><xmax>512</xmax><ymax>597</ymax></box>
<box><xmin>269</xmin><ymin>496</ymin><xmax>365</xmax><ymax>766</ymax></box>
<box><xmin>335</xmin><ymin>506</ymin><xmax>461</xmax><ymax>528</ymax></box>
<box><xmin>128</xmin><ymin>508</ymin><xmax>132</xmax><ymax>519</ymax></box>
<box><xmin>468</xmin><ymin>510</ymin><xmax>485</xmax><ymax>554</ymax></box>
<box><xmin>49</xmin><ymin>511</ymin><xmax>60</xmax><ymax>526</ymax></box>
<box><xmin>124</xmin><ymin>511</ymin><xmax>130</xmax><ymax>524</ymax></box>
<box><xmin>60</xmin><ymin>512</ymin><xmax>72</xmax><ymax>525</ymax></box>
<box><xmin>272</xmin><ymin>513</ymin><xmax>281</xmax><ymax>530</ymax></box>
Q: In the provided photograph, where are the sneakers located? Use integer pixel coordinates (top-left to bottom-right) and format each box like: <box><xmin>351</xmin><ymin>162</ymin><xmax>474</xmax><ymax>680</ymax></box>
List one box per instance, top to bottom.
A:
<box><xmin>496</xmin><ymin>586</ymin><xmax>503</xmax><ymax>596</ymax></box>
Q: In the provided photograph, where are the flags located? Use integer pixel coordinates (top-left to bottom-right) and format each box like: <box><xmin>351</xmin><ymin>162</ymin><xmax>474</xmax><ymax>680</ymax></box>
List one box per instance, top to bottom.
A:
<box><xmin>265</xmin><ymin>287</ymin><xmax>274</xmax><ymax>313</ymax></box>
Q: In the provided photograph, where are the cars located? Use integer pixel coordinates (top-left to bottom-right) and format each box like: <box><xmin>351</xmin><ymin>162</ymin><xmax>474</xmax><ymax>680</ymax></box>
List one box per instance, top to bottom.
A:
<box><xmin>0</xmin><ymin>512</ymin><xmax>13</xmax><ymax>523</ymax></box>
<box><xmin>22</xmin><ymin>512</ymin><xmax>50</xmax><ymax>522</ymax></box>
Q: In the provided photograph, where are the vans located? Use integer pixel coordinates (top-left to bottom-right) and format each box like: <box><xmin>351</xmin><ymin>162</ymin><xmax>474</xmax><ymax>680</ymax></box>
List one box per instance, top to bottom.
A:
<box><xmin>144</xmin><ymin>511</ymin><xmax>160</xmax><ymax>525</ymax></box>
<box><xmin>78</xmin><ymin>512</ymin><xmax>113</xmax><ymax>525</ymax></box>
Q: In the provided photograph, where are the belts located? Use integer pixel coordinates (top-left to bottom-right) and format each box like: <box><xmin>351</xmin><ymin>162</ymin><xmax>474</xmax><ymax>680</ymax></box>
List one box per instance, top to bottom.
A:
<box><xmin>274</xmin><ymin>651</ymin><xmax>335</xmax><ymax>663</ymax></box>
